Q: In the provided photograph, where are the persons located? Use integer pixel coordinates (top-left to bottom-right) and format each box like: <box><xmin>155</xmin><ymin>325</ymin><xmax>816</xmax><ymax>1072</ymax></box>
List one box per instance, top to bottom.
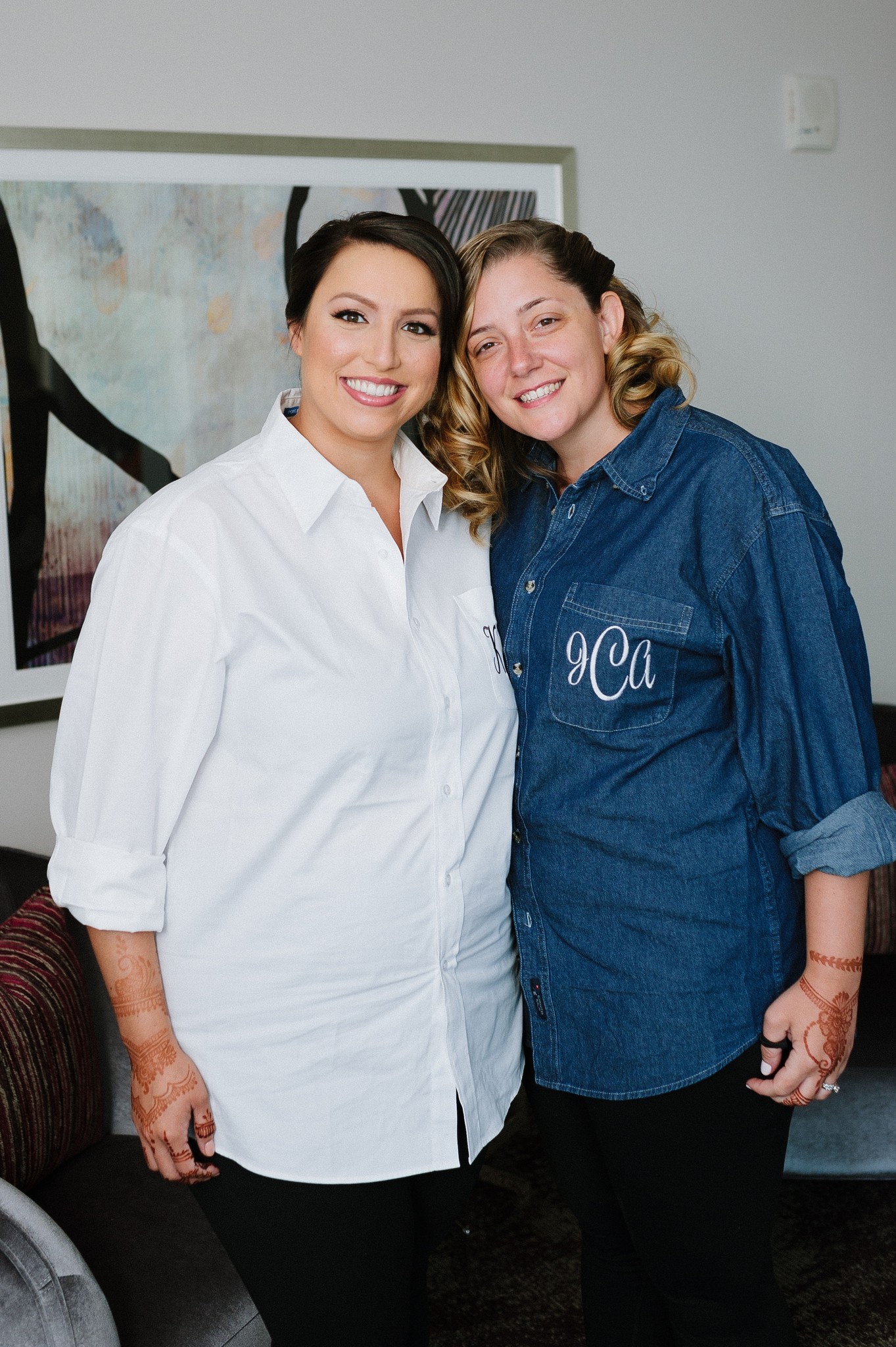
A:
<box><xmin>48</xmin><ymin>210</ymin><xmax>527</xmax><ymax>1347</ymax></box>
<box><xmin>418</xmin><ymin>220</ymin><xmax>896</xmax><ymax>1347</ymax></box>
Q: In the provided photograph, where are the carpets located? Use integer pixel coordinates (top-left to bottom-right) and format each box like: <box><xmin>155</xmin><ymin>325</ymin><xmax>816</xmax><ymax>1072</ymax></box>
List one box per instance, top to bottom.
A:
<box><xmin>779</xmin><ymin>1064</ymin><xmax>896</xmax><ymax>1180</ymax></box>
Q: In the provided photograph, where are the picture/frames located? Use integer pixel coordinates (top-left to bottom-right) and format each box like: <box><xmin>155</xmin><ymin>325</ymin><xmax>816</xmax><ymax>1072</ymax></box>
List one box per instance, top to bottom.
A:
<box><xmin>0</xmin><ymin>127</ymin><xmax>577</xmax><ymax>729</ymax></box>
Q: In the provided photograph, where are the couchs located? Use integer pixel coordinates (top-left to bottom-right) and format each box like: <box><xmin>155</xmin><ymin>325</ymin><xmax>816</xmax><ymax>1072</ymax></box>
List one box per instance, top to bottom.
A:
<box><xmin>0</xmin><ymin>846</ymin><xmax>105</xmax><ymax>1188</ymax></box>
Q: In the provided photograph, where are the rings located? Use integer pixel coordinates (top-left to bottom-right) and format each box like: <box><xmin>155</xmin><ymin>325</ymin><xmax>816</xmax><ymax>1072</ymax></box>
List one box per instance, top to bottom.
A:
<box><xmin>758</xmin><ymin>1033</ymin><xmax>786</xmax><ymax>1048</ymax></box>
<box><xmin>822</xmin><ymin>1083</ymin><xmax>840</xmax><ymax>1093</ymax></box>
